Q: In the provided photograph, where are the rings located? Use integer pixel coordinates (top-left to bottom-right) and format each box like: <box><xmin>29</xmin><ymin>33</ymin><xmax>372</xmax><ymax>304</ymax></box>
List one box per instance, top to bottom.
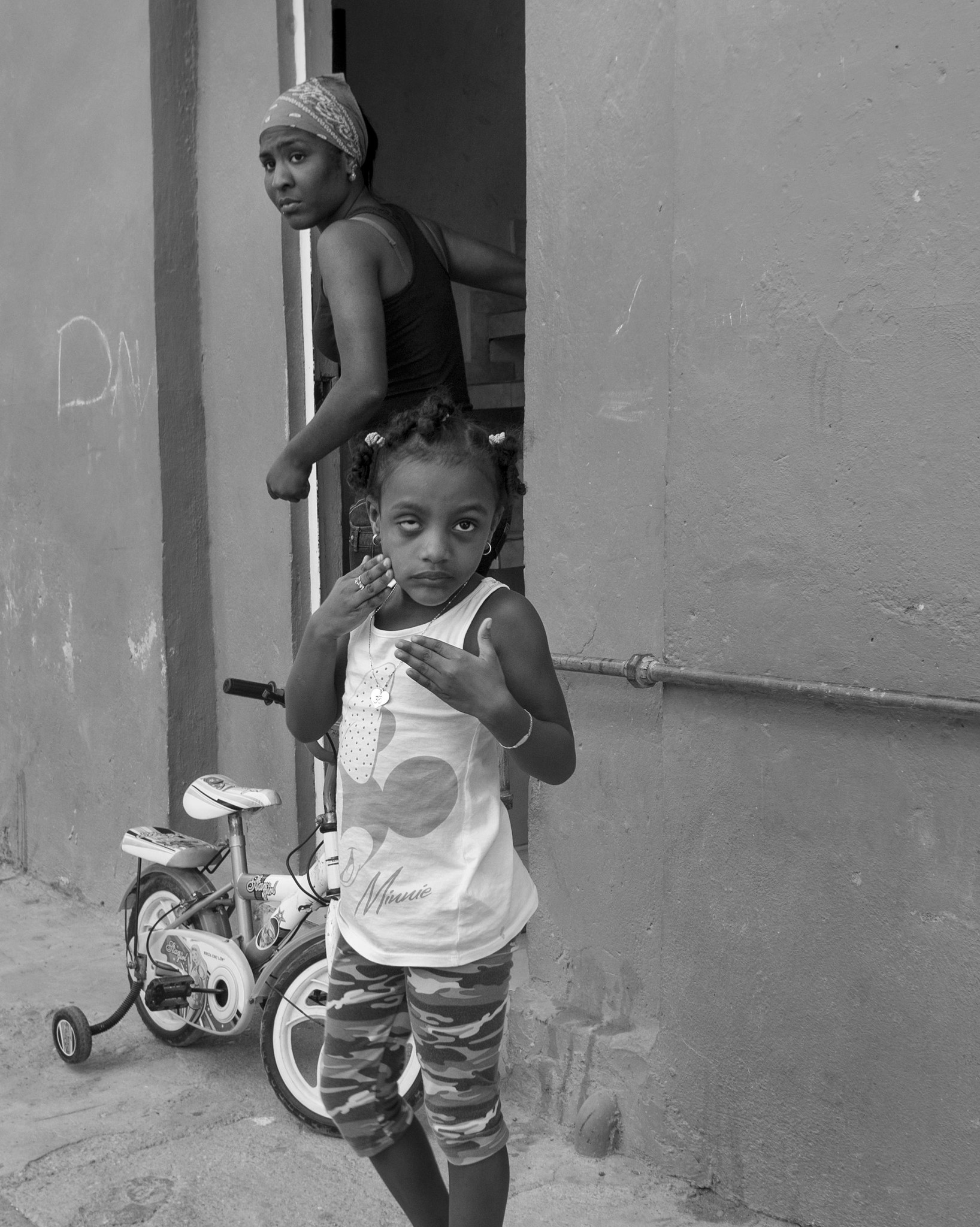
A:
<box><xmin>356</xmin><ymin>574</ymin><xmax>365</xmax><ymax>590</ymax></box>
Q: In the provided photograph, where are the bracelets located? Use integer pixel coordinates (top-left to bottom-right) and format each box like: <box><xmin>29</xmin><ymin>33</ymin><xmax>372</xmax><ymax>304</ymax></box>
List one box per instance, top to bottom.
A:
<box><xmin>499</xmin><ymin>708</ymin><xmax>533</xmax><ymax>749</ymax></box>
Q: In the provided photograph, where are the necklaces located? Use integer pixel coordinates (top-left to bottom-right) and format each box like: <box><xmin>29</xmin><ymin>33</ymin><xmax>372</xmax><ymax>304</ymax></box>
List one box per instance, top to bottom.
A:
<box><xmin>368</xmin><ymin>578</ymin><xmax>470</xmax><ymax>707</ymax></box>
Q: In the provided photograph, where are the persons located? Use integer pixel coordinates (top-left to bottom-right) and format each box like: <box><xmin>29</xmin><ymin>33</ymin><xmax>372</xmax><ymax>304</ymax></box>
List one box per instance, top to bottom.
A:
<box><xmin>259</xmin><ymin>73</ymin><xmax>526</xmax><ymax>500</ymax></box>
<box><xmin>284</xmin><ymin>395</ymin><xmax>575</xmax><ymax>1227</ymax></box>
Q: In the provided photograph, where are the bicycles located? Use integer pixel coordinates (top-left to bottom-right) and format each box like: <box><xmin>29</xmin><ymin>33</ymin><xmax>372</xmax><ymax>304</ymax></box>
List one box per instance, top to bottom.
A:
<box><xmin>50</xmin><ymin>677</ymin><xmax>424</xmax><ymax>1140</ymax></box>
